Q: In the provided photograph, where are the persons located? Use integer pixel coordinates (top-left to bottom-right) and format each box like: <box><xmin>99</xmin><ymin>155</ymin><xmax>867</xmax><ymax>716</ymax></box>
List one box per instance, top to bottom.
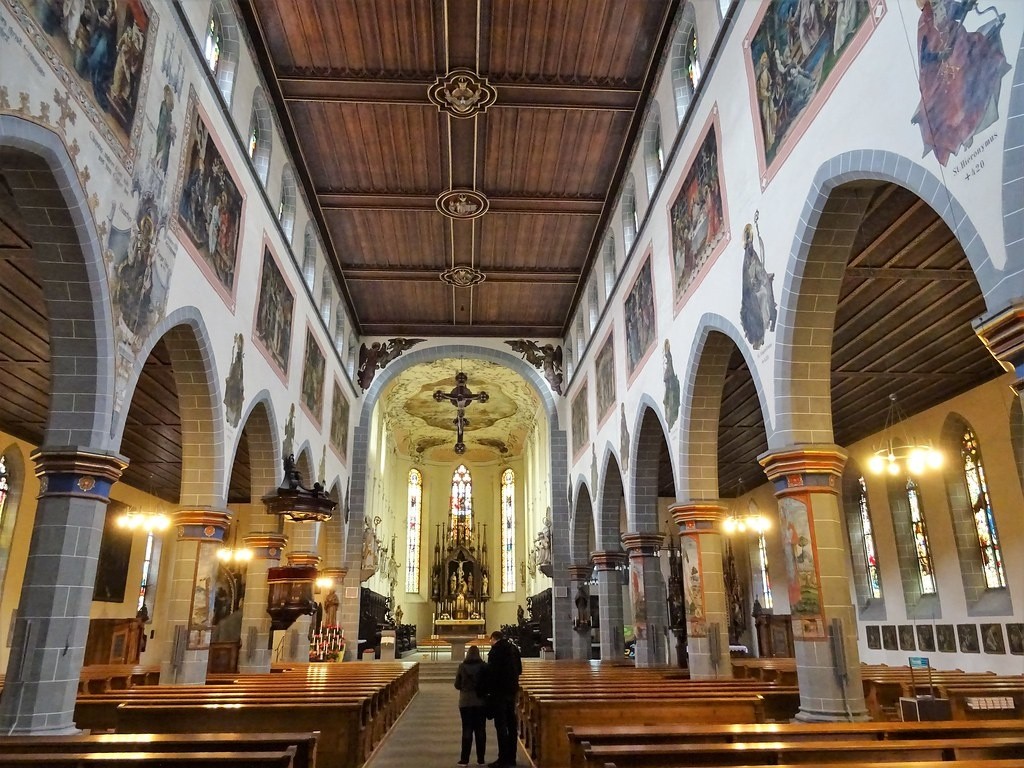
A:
<box><xmin>440</xmin><ymin>393</ymin><xmax>480</xmax><ymax>436</ymax></box>
<box><xmin>365</xmin><ymin>527</ymin><xmax>379</xmax><ymax>566</ymax></box>
<box><xmin>537</xmin><ymin>532</ymin><xmax>549</xmax><ymax>564</ymax></box>
<box><xmin>390</xmin><ymin>554</ymin><xmax>401</xmax><ymax>587</ymax></box>
<box><xmin>454</xmin><ymin>560</ymin><xmax>468</xmax><ymax>576</ymax></box>
<box><xmin>450</xmin><ymin>572</ymin><xmax>456</xmax><ymax>591</ymax></box>
<box><xmin>468</xmin><ymin>572</ymin><xmax>472</xmax><ymax>591</ymax></box>
<box><xmin>483</xmin><ymin>574</ymin><xmax>488</xmax><ymax>593</ymax></box>
<box><xmin>434</xmin><ymin>575</ymin><xmax>440</xmax><ymax>593</ymax></box>
<box><xmin>575</xmin><ymin>585</ymin><xmax>589</xmax><ymax>621</ymax></box>
<box><xmin>324</xmin><ymin>587</ymin><xmax>339</xmax><ymax>626</ymax></box>
<box><xmin>396</xmin><ymin>605</ymin><xmax>403</xmax><ymax>628</ymax></box>
<box><xmin>517</xmin><ymin>605</ymin><xmax>524</xmax><ymax>619</ymax></box>
<box><xmin>487</xmin><ymin>631</ymin><xmax>523</xmax><ymax>768</ymax></box>
<box><xmin>454</xmin><ymin>645</ymin><xmax>488</xmax><ymax>767</ymax></box>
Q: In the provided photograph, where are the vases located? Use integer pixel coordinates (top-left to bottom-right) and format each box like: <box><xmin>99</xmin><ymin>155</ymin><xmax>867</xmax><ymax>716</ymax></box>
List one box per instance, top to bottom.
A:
<box><xmin>363</xmin><ymin>652</ymin><xmax>375</xmax><ymax>662</ymax></box>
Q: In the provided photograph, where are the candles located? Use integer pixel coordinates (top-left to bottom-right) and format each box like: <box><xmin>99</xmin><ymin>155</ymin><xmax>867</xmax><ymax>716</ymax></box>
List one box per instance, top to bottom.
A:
<box><xmin>432</xmin><ymin>598</ymin><xmax>485</xmax><ymax>623</ymax></box>
<box><xmin>321</xmin><ymin>621</ymin><xmax>322</xmax><ymax>632</ymax></box>
<box><xmin>337</xmin><ymin>621</ymin><xmax>339</xmax><ymax>632</ymax></box>
<box><xmin>327</xmin><ymin>628</ymin><xmax>328</xmax><ymax>636</ymax></box>
<box><xmin>319</xmin><ymin>629</ymin><xmax>321</xmax><ymax>637</ymax></box>
<box><xmin>334</xmin><ymin>629</ymin><xmax>336</xmax><ymax>637</ymax></box>
<box><xmin>312</xmin><ymin>630</ymin><xmax>314</xmax><ymax>638</ymax></box>
<box><xmin>341</xmin><ymin>630</ymin><xmax>344</xmax><ymax>638</ymax></box>
<box><xmin>320</xmin><ymin>634</ymin><xmax>322</xmax><ymax>643</ymax></box>
<box><xmin>328</xmin><ymin>634</ymin><xmax>330</xmax><ymax>644</ymax></box>
<box><xmin>336</xmin><ymin>635</ymin><xmax>339</xmax><ymax>645</ymax></box>
<box><xmin>314</xmin><ymin>637</ymin><xmax>315</xmax><ymax>644</ymax></box>
<box><xmin>340</xmin><ymin>638</ymin><xmax>342</xmax><ymax>647</ymax></box>
<box><xmin>331</xmin><ymin>639</ymin><xmax>334</xmax><ymax>650</ymax></box>
<box><xmin>317</xmin><ymin>640</ymin><xmax>319</xmax><ymax>649</ymax></box>
<box><xmin>324</xmin><ymin>640</ymin><xmax>325</xmax><ymax>650</ymax></box>
<box><xmin>339</xmin><ymin>642</ymin><xmax>341</xmax><ymax>650</ymax></box>
<box><xmin>326</xmin><ymin>644</ymin><xmax>327</xmax><ymax>654</ymax></box>
<box><xmin>318</xmin><ymin>645</ymin><xmax>319</xmax><ymax>655</ymax></box>
<box><xmin>320</xmin><ymin>651</ymin><xmax>324</xmax><ymax>660</ymax></box>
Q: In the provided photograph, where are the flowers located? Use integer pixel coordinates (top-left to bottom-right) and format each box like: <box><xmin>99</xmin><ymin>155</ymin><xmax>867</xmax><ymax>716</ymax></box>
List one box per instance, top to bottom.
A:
<box><xmin>309</xmin><ymin>624</ymin><xmax>345</xmax><ymax>662</ymax></box>
<box><xmin>508</xmin><ymin>638</ymin><xmax>521</xmax><ymax>652</ymax></box>
<box><xmin>363</xmin><ymin>649</ymin><xmax>374</xmax><ymax>653</ymax></box>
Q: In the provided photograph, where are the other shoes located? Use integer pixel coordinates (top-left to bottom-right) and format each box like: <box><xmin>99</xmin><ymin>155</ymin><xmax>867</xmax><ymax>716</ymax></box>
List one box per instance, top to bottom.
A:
<box><xmin>488</xmin><ymin>758</ymin><xmax>516</xmax><ymax>768</ymax></box>
<box><xmin>457</xmin><ymin>761</ymin><xmax>468</xmax><ymax>767</ymax></box>
<box><xmin>476</xmin><ymin>762</ymin><xmax>485</xmax><ymax>766</ymax></box>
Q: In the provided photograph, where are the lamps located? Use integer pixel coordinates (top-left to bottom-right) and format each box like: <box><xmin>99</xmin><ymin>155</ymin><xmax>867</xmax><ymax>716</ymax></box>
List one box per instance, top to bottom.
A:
<box><xmin>868</xmin><ymin>392</ymin><xmax>944</xmax><ymax>475</ymax></box>
<box><xmin>116</xmin><ymin>472</ymin><xmax>172</xmax><ymax>532</ymax></box>
<box><xmin>725</xmin><ymin>475</ymin><xmax>770</xmax><ymax>535</ymax></box>
<box><xmin>215</xmin><ymin>504</ymin><xmax>253</xmax><ymax>562</ymax></box>
<box><xmin>653</xmin><ymin>521</ymin><xmax>683</xmax><ymax>558</ymax></box>
<box><xmin>616</xmin><ymin>552</ymin><xmax>631</xmax><ymax>571</ymax></box>
<box><xmin>583</xmin><ymin>566</ymin><xmax>599</xmax><ymax>586</ymax></box>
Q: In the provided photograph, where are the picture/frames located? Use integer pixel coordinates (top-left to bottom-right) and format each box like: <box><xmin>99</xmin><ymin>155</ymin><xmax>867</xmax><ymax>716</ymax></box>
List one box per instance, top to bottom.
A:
<box><xmin>345</xmin><ymin>587</ymin><xmax>359</xmax><ymax>599</ymax></box>
<box><xmin>980</xmin><ymin>623</ymin><xmax>1006</xmax><ymax>653</ymax></box>
<box><xmin>1005</xmin><ymin>623</ymin><xmax>1024</xmax><ymax>655</ymax></box>
<box><xmin>898</xmin><ymin>624</ymin><xmax>915</xmax><ymax>651</ymax></box>
<box><xmin>916</xmin><ymin>624</ymin><xmax>935</xmax><ymax>652</ymax></box>
<box><xmin>957</xmin><ymin>624</ymin><xmax>980</xmax><ymax>653</ymax></box>
<box><xmin>865</xmin><ymin>625</ymin><xmax>882</xmax><ymax>649</ymax></box>
<box><xmin>881</xmin><ymin>625</ymin><xmax>898</xmax><ymax>650</ymax></box>
<box><xmin>936</xmin><ymin>625</ymin><xmax>956</xmax><ymax>653</ymax></box>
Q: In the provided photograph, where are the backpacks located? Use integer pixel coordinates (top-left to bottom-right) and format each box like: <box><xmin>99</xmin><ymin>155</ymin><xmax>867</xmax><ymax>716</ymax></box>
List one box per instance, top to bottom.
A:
<box><xmin>480</xmin><ymin>686</ymin><xmax>501</xmax><ymax>719</ymax></box>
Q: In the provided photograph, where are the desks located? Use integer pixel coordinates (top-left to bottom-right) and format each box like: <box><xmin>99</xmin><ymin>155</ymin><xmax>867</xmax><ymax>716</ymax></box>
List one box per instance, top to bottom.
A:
<box><xmin>436</xmin><ymin>619</ymin><xmax>486</xmax><ymax>660</ymax></box>
<box><xmin>900</xmin><ymin>697</ymin><xmax>951</xmax><ymax>722</ymax></box>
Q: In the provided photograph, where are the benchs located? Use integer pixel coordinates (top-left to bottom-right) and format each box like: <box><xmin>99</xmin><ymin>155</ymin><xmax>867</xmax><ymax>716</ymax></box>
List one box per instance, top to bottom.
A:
<box><xmin>0</xmin><ymin>660</ymin><xmax>1024</xmax><ymax>768</ymax></box>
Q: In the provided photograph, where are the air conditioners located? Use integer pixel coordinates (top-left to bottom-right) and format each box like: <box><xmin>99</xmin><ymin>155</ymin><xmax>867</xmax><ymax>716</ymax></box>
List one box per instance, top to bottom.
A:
<box><xmin>555</xmin><ymin>587</ymin><xmax>567</xmax><ymax>598</ymax></box>
